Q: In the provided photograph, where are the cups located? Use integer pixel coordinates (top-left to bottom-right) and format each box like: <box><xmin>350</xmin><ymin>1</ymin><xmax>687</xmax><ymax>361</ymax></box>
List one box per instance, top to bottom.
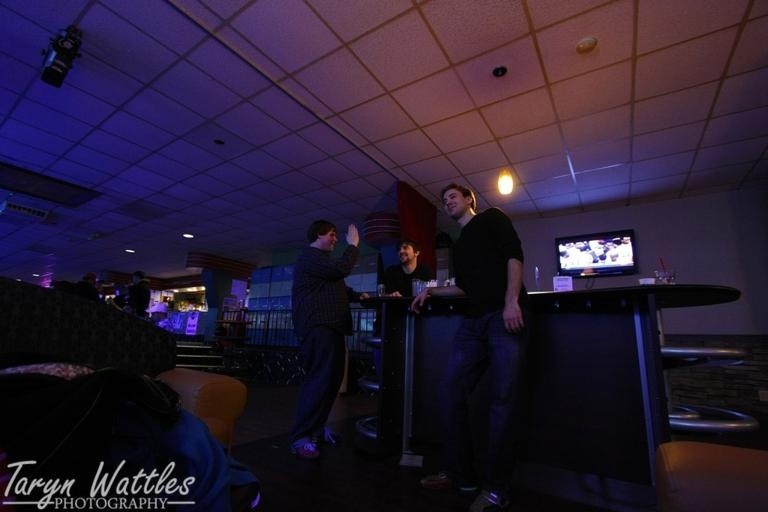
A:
<box><xmin>423</xmin><ymin>275</ymin><xmax>455</xmax><ymax>288</ymax></box>
<box><xmin>412</xmin><ymin>278</ymin><xmax>420</xmax><ymax>296</ymax></box>
<box><xmin>378</xmin><ymin>284</ymin><xmax>385</xmax><ymax>296</ymax></box>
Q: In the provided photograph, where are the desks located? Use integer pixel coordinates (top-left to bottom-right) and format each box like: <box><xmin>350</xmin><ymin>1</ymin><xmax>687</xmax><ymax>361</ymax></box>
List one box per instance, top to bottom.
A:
<box><xmin>213</xmin><ymin>317</ymin><xmax>248</xmax><ymax>351</ymax></box>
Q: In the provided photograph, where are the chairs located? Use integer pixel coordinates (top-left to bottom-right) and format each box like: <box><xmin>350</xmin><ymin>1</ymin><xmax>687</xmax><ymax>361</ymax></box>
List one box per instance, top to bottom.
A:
<box><xmin>142</xmin><ymin>364</ymin><xmax>246</xmax><ymax>464</ymax></box>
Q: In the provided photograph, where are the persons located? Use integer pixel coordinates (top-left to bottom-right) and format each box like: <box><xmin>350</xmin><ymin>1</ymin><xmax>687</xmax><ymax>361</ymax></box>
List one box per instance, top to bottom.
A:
<box><xmin>411</xmin><ymin>182</ymin><xmax>525</xmax><ymax>512</ymax></box>
<box><xmin>290</xmin><ymin>218</ymin><xmax>369</xmax><ymax>459</ymax></box>
<box><xmin>558</xmin><ymin>236</ymin><xmax>634</xmax><ymax>269</ymax></box>
<box><xmin>377</xmin><ymin>239</ymin><xmax>431</xmax><ymax>298</ymax></box>
<box><xmin>64</xmin><ymin>271</ymin><xmax>150</xmax><ymax>320</ymax></box>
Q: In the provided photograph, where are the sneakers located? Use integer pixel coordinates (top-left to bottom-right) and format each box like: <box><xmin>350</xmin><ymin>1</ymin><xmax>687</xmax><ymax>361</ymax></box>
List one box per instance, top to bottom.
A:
<box><xmin>314</xmin><ymin>425</ymin><xmax>341</xmax><ymax>446</ymax></box>
<box><xmin>289</xmin><ymin>436</ymin><xmax>319</xmax><ymax>460</ymax></box>
<box><xmin>418</xmin><ymin>469</ymin><xmax>480</xmax><ymax>492</ymax></box>
<box><xmin>470</xmin><ymin>484</ymin><xmax>511</xmax><ymax>511</ymax></box>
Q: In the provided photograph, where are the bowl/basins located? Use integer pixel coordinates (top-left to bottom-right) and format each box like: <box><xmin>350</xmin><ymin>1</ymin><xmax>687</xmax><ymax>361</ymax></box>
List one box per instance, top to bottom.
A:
<box><xmin>654</xmin><ymin>271</ymin><xmax>684</xmax><ymax>285</ymax></box>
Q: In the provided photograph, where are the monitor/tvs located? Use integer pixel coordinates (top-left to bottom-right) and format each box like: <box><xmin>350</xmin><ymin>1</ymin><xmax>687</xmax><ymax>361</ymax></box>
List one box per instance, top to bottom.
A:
<box><xmin>555</xmin><ymin>229</ymin><xmax>640</xmax><ymax>278</ymax></box>
